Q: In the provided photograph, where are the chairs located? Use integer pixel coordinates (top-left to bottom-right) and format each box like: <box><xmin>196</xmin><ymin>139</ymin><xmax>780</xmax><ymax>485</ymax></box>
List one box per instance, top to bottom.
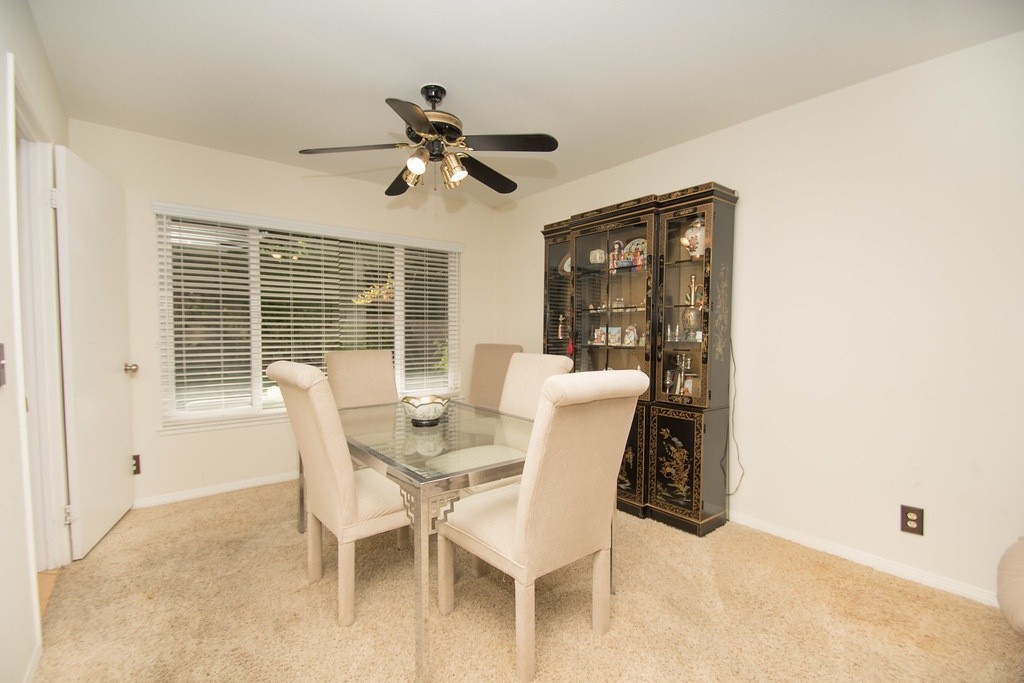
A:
<box><xmin>265</xmin><ymin>343</ymin><xmax>651</xmax><ymax>683</ymax></box>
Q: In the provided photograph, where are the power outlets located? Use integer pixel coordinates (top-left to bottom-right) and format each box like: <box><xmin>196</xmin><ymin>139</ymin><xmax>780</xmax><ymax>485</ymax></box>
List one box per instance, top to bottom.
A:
<box><xmin>133</xmin><ymin>455</ymin><xmax>140</xmax><ymax>474</ymax></box>
<box><xmin>900</xmin><ymin>505</ymin><xmax>923</xmax><ymax>536</ymax></box>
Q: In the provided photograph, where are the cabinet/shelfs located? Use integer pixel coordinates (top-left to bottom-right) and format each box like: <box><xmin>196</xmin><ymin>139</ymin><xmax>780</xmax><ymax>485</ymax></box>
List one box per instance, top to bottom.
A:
<box><xmin>541</xmin><ymin>181</ymin><xmax>737</xmax><ymax>537</ymax></box>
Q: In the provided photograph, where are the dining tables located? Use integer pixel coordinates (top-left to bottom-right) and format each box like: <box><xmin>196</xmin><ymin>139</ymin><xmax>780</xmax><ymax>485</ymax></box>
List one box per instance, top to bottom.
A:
<box><xmin>297</xmin><ymin>394</ymin><xmax>616</xmax><ymax>683</ymax></box>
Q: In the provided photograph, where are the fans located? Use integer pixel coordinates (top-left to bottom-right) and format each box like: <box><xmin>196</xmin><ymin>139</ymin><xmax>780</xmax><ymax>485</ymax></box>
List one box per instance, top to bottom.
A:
<box><xmin>299</xmin><ymin>85</ymin><xmax>559</xmax><ymax>196</ymax></box>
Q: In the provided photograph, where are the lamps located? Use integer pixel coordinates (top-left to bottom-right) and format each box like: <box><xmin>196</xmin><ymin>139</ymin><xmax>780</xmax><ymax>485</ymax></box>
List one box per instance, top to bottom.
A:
<box><xmin>402</xmin><ymin>139</ymin><xmax>468</xmax><ymax>190</ymax></box>
